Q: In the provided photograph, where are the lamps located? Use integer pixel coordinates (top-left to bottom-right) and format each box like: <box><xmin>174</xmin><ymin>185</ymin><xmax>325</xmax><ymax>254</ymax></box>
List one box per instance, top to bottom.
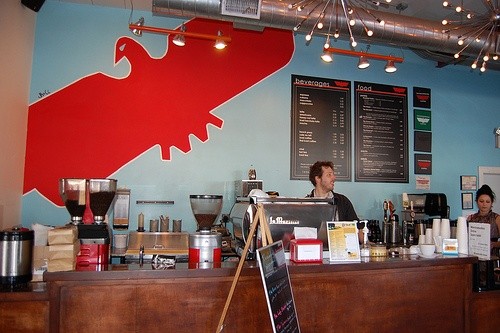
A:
<box><xmin>288</xmin><ymin>0</ymin><xmax>392</xmax><ymax>51</ymax></box>
<box><xmin>441</xmin><ymin>0</ymin><xmax>500</xmax><ymax>72</ymax></box>
<box><xmin>130</xmin><ymin>18</ymin><xmax>145</xmax><ymax>36</ymax></box>
<box><xmin>172</xmin><ymin>24</ymin><xmax>189</xmax><ymax>46</ymax></box>
<box><xmin>213</xmin><ymin>29</ymin><xmax>227</xmax><ymax>50</ymax></box>
<box><xmin>320</xmin><ymin>51</ymin><xmax>334</xmax><ymax>63</ymax></box>
<box><xmin>358</xmin><ymin>55</ymin><xmax>369</xmax><ymax>69</ymax></box>
<box><xmin>385</xmin><ymin>55</ymin><xmax>396</xmax><ymax>73</ymax></box>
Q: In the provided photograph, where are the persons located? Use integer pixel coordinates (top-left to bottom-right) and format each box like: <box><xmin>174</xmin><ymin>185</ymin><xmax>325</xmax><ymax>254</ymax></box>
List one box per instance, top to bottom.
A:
<box><xmin>304</xmin><ymin>160</ymin><xmax>377</xmax><ymax>249</ymax></box>
<box><xmin>467</xmin><ymin>184</ymin><xmax>500</xmax><ymax>284</ymax></box>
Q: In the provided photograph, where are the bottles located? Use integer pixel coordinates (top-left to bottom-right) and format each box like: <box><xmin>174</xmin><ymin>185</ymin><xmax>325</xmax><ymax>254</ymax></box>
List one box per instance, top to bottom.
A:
<box><xmin>249</xmin><ymin>164</ymin><xmax>256</xmax><ymax>179</ymax></box>
<box><xmin>138</xmin><ymin>213</ymin><xmax>144</xmax><ymax>232</ymax></box>
<box><xmin>367</xmin><ymin>220</ymin><xmax>381</xmax><ymax>244</ymax></box>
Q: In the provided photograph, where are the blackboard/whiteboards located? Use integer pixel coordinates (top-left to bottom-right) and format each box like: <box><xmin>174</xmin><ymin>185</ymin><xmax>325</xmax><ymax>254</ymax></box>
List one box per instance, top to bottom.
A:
<box><xmin>256</xmin><ymin>240</ymin><xmax>301</xmax><ymax>333</ymax></box>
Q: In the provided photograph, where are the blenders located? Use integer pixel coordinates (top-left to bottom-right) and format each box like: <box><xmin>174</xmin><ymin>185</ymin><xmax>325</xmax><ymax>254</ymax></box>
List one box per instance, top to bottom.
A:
<box><xmin>60</xmin><ymin>178</ymin><xmax>118</xmax><ymax>272</ymax></box>
<box><xmin>110</xmin><ymin>189</ymin><xmax>130</xmax><ymax>265</ymax></box>
<box><xmin>189</xmin><ymin>195</ymin><xmax>223</xmax><ymax>270</ymax></box>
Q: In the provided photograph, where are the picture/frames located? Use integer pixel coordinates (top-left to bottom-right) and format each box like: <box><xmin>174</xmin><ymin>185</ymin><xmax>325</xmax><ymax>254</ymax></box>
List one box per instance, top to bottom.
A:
<box><xmin>412</xmin><ymin>87</ymin><xmax>433</xmax><ymax>108</ymax></box>
<box><xmin>415</xmin><ymin>110</ymin><xmax>432</xmax><ymax>131</ymax></box>
<box><xmin>413</xmin><ymin>132</ymin><xmax>432</xmax><ymax>152</ymax></box>
<box><xmin>413</xmin><ymin>153</ymin><xmax>434</xmax><ymax>175</ymax></box>
<box><xmin>461</xmin><ymin>175</ymin><xmax>478</xmax><ymax>191</ymax></box>
<box><xmin>462</xmin><ymin>193</ymin><xmax>474</xmax><ymax>210</ymax></box>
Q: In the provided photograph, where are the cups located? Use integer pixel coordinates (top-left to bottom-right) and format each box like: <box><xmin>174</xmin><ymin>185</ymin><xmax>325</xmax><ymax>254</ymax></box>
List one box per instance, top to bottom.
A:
<box><xmin>456</xmin><ymin>217</ymin><xmax>468</xmax><ymax>255</ymax></box>
<box><xmin>160</xmin><ymin>219</ymin><xmax>170</xmax><ymax>232</ymax></box>
<box><xmin>432</xmin><ymin>219</ymin><xmax>441</xmax><ymax>244</ymax></box>
<box><xmin>441</xmin><ymin>219</ymin><xmax>451</xmax><ymax>239</ymax></box>
<box><xmin>150</xmin><ymin>220</ymin><xmax>158</xmax><ymax>232</ymax></box>
<box><xmin>173</xmin><ymin>220</ymin><xmax>181</xmax><ymax>232</ymax></box>
<box><xmin>425</xmin><ymin>229</ymin><xmax>432</xmax><ymax>244</ymax></box>
<box><xmin>419</xmin><ymin>235</ymin><xmax>425</xmax><ymax>244</ymax></box>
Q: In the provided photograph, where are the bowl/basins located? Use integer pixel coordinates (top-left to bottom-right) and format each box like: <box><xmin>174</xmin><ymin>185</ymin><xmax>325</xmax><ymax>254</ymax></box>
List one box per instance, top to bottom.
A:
<box><xmin>420</xmin><ymin>244</ymin><xmax>435</xmax><ymax>256</ymax></box>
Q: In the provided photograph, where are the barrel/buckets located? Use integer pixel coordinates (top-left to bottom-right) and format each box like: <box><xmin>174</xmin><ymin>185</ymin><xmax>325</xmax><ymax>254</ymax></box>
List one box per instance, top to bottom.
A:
<box><xmin>0</xmin><ymin>226</ymin><xmax>35</xmax><ymax>292</ymax></box>
